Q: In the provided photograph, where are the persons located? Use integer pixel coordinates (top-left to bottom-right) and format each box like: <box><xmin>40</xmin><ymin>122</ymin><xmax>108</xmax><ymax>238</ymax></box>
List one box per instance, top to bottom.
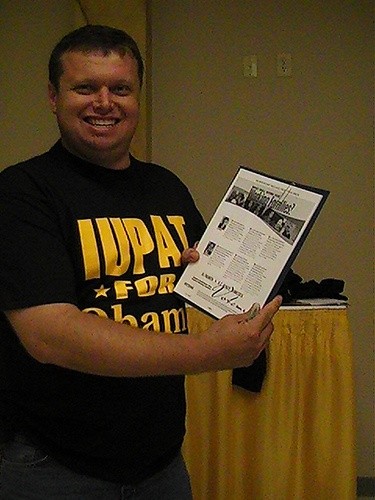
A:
<box><xmin>0</xmin><ymin>24</ymin><xmax>282</xmax><ymax>499</ymax></box>
<box><xmin>204</xmin><ymin>188</ymin><xmax>293</xmax><ymax>263</ymax></box>
